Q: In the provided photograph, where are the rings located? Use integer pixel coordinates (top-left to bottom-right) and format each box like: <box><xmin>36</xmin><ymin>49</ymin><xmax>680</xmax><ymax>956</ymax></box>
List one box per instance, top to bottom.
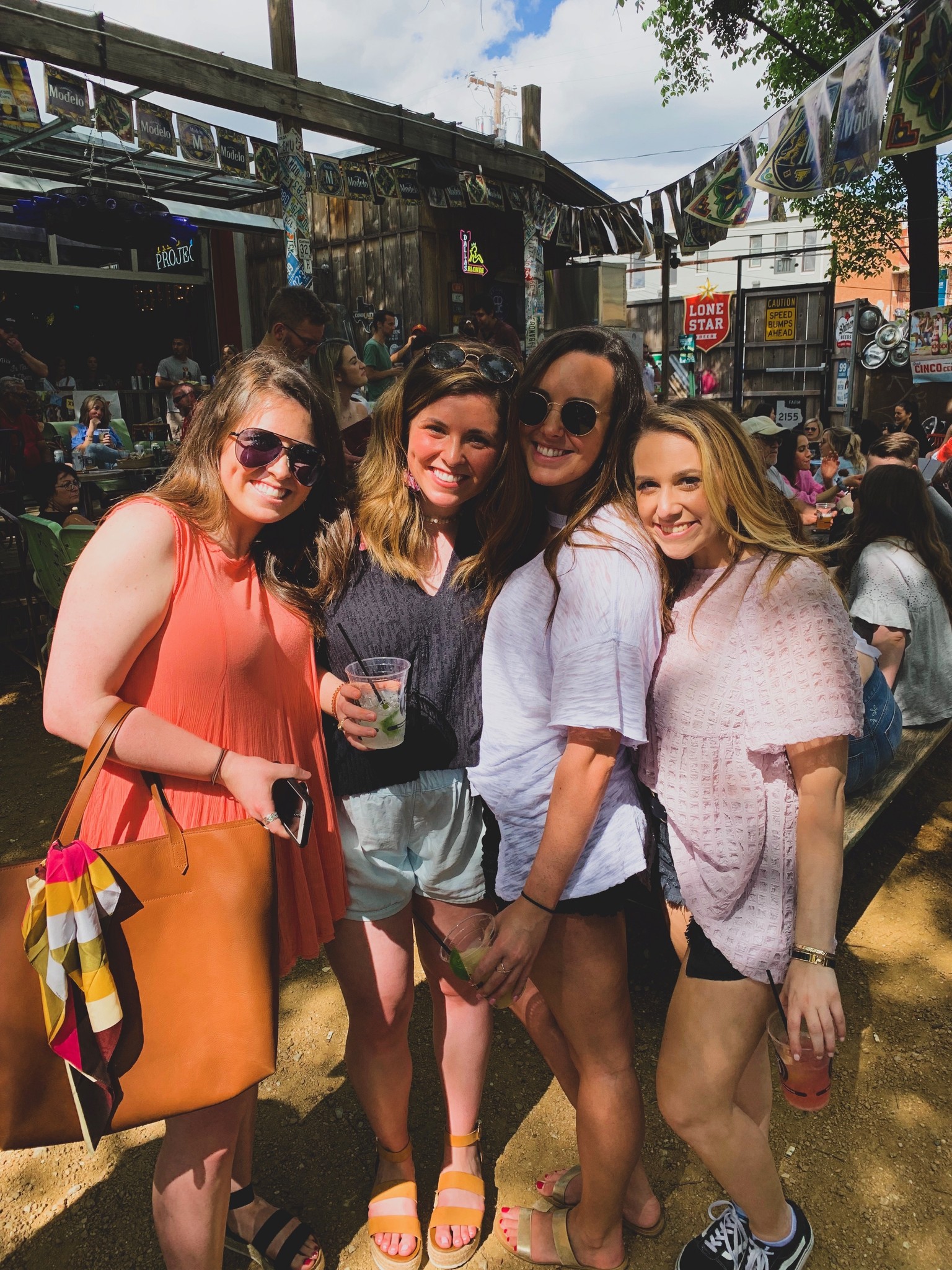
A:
<box><xmin>46</xmin><ymin>442</ymin><xmax>48</xmax><ymax>444</ymax></box>
<box><xmin>48</xmin><ymin>448</ymin><xmax>50</xmax><ymax>450</ymax></box>
<box><xmin>337</xmin><ymin>717</ymin><xmax>356</xmax><ymax>731</ymax></box>
<box><xmin>292</xmin><ymin>809</ymin><xmax>302</xmax><ymax>817</ymax></box>
<box><xmin>262</xmin><ymin>811</ymin><xmax>279</xmax><ymax>830</ymax></box>
<box><xmin>496</xmin><ymin>960</ymin><xmax>512</xmax><ymax>977</ymax></box>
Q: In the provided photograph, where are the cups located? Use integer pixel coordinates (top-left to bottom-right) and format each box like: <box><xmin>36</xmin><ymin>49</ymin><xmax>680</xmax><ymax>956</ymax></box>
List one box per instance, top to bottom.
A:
<box><xmin>75</xmin><ymin>379</ymin><xmax>84</xmax><ymax>391</ymax></box>
<box><xmin>97</xmin><ymin>379</ymin><xmax>106</xmax><ymax>390</ymax></box>
<box><xmin>115</xmin><ymin>379</ymin><xmax>122</xmax><ymax>390</ymax></box>
<box><xmin>97</xmin><ymin>429</ymin><xmax>110</xmax><ymax>444</ymax></box>
<box><xmin>118</xmin><ymin>450</ymin><xmax>130</xmax><ymax>460</ymax></box>
<box><xmin>82</xmin><ymin>454</ymin><xmax>95</xmax><ymax>470</ymax></box>
<box><xmin>815</xmin><ymin>502</ymin><xmax>835</xmax><ymax>529</ymax></box>
<box><xmin>344</xmin><ymin>657</ymin><xmax>411</xmax><ymax>749</ymax></box>
<box><xmin>439</xmin><ymin>912</ymin><xmax>526</xmax><ymax>1009</ymax></box>
<box><xmin>766</xmin><ymin>1008</ymin><xmax>833</xmax><ymax>1111</ymax></box>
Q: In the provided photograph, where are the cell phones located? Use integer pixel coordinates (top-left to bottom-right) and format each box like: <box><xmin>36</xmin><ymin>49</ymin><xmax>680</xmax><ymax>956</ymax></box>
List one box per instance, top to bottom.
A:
<box><xmin>271</xmin><ymin>760</ymin><xmax>313</xmax><ymax>847</ymax></box>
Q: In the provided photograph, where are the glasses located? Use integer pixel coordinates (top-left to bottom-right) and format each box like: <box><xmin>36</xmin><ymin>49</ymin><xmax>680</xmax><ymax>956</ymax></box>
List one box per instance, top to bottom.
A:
<box><xmin>282</xmin><ymin>323</ymin><xmax>328</xmax><ymax>350</ymax></box>
<box><xmin>4</xmin><ymin>328</ymin><xmax>19</xmax><ymax>334</ymax></box>
<box><xmin>423</xmin><ymin>341</ymin><xmax>520</xmax><ymax>387</ymax></box>
<box><xmin>519</xmin><ymin>391</ymin><xmax>613</xmax><ymax>437</ymax></box>
<box><xmin>173</xmin><ymin>393</ymin><xmax>190</xmax><ymax>404</ymax></box>
<box><xmin>26</xmin><ymin>409</ymin><xmax>42</xmax><ymax>415</ymax></box>
<box><xmin>805</xmin><ymin>426</ymin><xmax>817</xmax><ymax>431</ymax></box>
<box><xmin>227</xmin><ymin>427</ymin><xmax>325</xmax><ymax>488</ymax></box>
<box><xmin>751</xmin><ymin>435</ymin><xmax>784</xmax><ymax>447</ymax></box>
<box><xmin>820</xmin><ymin>437</ymin><xmax>826</xmax><ymax>444</ymax></box>
<box><xmin>54</xmin><ymin>481</ymin><xmax>82</xmax><ymax>491</ymax></box>
<box><xmin>851</xmin><ymin>487</ymin><xmax>859</xmax><ymax>502</ymax></box>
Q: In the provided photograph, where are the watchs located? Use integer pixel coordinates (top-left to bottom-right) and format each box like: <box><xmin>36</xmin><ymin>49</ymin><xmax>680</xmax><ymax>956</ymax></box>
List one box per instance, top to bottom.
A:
<box><xmin>20</xmin><ymin>349</ymin><xmax>25</xmax><ymax>355</ymax></box>
<box><xmin>113</xmin><ymin>443</ymin><xmax>116</xmax><ymax>448</ymax></box>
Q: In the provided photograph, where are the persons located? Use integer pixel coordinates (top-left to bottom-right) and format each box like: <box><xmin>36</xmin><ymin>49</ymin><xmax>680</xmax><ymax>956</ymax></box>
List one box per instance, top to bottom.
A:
<box><xmin>156</xmin><ymin>285</ymin><xmax>522</xmax><ymax>476</ymax></box>
<box><xmin>912</xmin><ymin>312</ymin><xmax>952</xmax><ymax>350</ymax></box>
<box><xmin>0</xmin><ymin>314</ymin><xmax>155</xmax><ymax>391</ymax></box>
<box><xmin>452</xmin><ymin>325</ymin><xmax>671</xmax><ymax>1270</ymax></box>
<box><xmin>317</xmin><ymin>335</ymin><xmax>526</xmax><ymax>1270</ymax></box>
<box><xmin>642</xmin><ymin>344</ymin><xmax>952</xmax><ymax>795</ymax></box>
<box><xmin>43</xmin><ymin>353</ymin><xmax>356</xmax><ymax>1270</ymax></box>
<box><xmin>0</xmin><ymin>376</ymin><xmax>54</xmax><ymax>540</ymax></box>
<box><xmin>26</xmin><ymin>390</ymin><xmax>117</xmax><ymax>509</ymax></box>
<box><xmin>68</xmin><ymin>394</ymin><xmax>151</xmax><ymax>493</ymax></box>
<box><xmin>611</xmin><ymin>398</ymin><xmax>865</xmax><ymax>1270</ymax></box>
<box><xmin>46</xmin><ymin>406</ymin><xmax>63</xmax><ymax>422</ymax></box>
<box><xmin>37</xmin><ymin>462</ymin><xmax>101</xmax><ymax>528</ymax></box>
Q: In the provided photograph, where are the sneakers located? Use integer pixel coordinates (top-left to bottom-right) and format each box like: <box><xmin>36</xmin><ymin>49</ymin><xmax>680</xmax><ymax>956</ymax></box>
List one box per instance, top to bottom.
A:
<box><xmin>738</xmin><ymin>1199</ymin><xmax>814</xmax><ymax>1270</ymax></box>
<box><xmin>675</xmin><ymin>1200</ymin><xmax>750</xmax><ymax>1270</ymax></box>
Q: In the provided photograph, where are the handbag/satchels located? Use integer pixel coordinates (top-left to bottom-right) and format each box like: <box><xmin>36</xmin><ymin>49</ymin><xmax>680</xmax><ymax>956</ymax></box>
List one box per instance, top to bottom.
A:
<box><xmin>0</xmin><ymin>700</ymin><xmax>276</xmax><ymax>1151</ymax></box>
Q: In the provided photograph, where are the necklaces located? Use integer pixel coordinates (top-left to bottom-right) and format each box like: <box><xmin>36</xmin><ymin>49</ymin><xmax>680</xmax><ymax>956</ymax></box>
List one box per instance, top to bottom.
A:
<box><xmin>342</xmin><ymin>406</ymin><xmax>352</xmax><ymax>421</ymax></box>
<box><xmin>0</xmin><ymin>408</ymin><xmax>18</xmax><ymax>423</ymax></box>
<box><xmin>419</xmin><ymin>511</ymin><xmax>462</xmax><ymax>524</ymax></box>
<box><xmin>210</xmin><ymin>517</ymin><xmax>241</xmax><ymax>559</ymax></box>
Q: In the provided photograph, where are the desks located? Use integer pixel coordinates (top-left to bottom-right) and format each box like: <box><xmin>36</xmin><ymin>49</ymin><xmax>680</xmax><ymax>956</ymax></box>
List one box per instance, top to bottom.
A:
<box><xmin>77</xmin><ymin>465</ymin><xmax>169</xmax><ymax>523</ymax></box>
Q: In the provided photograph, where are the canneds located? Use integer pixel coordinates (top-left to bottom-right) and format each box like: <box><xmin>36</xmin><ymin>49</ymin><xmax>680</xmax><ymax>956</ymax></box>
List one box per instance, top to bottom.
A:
<box><xmin>130</xmin><ymin>375</ymin><xmax>143</xmax><ymax>390</ymax></box>
<box><xmin>145</xmin><ymin>375</ymin><xmax>153</xmax><ymax>389</ymax></box>
<box><xmin>200</xmin><ymin>375</ymin><xmax>207</xmax><ymax>385</ymax></box>
<box><xmin>151</xmin><ymin>443</ymin><xmax>163</xmax><ymax>467</ymax></box>
<box><xmin>134</xmin><ymin>444</ymin><xmax>145</xmax><ymax>455</ymax></box>
<box><xmin>54</xmin><ymin>449</ymin><xmax>64</xmax><ymax>464</ymax></box>
<box><xmin>71</xmin><ymin>449</ymin><xmax>84</xmax><ymax>471</ymax></box>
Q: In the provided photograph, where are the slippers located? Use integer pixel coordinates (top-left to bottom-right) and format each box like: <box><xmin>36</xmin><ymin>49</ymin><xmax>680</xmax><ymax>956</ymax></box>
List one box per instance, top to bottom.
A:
<box><xmin>535</xmin><ymin>1164</ymin><xmax>666</xmax><ymax>1238</ymax></box>
<box><xmin>494</xmin><ymin>1206</ymin><xmax>629</xmax><ymax>1270</ymax></box>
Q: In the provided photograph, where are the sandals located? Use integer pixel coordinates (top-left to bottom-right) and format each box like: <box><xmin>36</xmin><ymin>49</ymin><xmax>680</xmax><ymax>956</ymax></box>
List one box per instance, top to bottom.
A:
<box><xmin>426</xmin><ymin>1119</ymin><xmax>485</xmax><ymax>1270</ymax></box>
<box><xmin>368</xmin><ymin>1132</ymin><xmax>422</xmax><ymax>1270</ymax></box>
<box><xmin>223</xmin><ymin>1179</ymin><xmax>325</xmax><ymax>1270</ymax></box>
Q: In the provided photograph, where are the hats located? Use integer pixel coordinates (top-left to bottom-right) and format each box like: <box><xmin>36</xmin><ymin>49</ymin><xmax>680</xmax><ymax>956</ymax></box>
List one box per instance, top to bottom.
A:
<box><xmin>0</xmin><ymin>316</ymin><xmax>22</xmax><ymax>335</ymax></box>
<box><xmin>466</xmin><ymin>320</ymin><xmax>472</xmax><ymax>324</ymax></box>
<box><xmin>412</xmin><ymin>324</ymin><xmax>427</xmax><ymax>337</ymax></box>
<box><xmin>741</xmin><ymin>416</ymin><xmax>792</xmax><ymax>440</ymax></box>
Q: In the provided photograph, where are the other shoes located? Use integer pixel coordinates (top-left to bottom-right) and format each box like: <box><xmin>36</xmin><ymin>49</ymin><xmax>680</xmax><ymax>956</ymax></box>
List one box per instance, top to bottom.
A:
<box><xmin>100</xmin><ymin>497</ymin><xmax>121</xmax><ymax>508</ymax></box>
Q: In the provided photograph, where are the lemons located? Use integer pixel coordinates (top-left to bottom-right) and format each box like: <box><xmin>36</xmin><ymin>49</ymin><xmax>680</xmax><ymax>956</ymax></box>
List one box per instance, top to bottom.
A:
<box><xmin>379</xmin><ymin>708</ymin><xmax>403</xmax><ymax>737</ymax></box>
<box><xmin>449</xmin><ymin>947</ymin><xmax>472</xmax><ymax>981</ymax></box>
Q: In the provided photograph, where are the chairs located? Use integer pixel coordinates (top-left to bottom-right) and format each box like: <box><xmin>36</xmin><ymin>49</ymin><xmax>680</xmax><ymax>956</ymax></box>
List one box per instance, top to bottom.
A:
<box><xmin>47</xmin><ymin>418</ymin><xmax>135</xmax><ymax>522</ymax></box>
<box><xmin>0</xmin><ymin>428</ymin><xmax>98</xmax><ymax>692</ymax></box>
<box><xmin>926</xmin><ymin>434</ymin><xmax>946</xmax><ymax>450</ymax></box>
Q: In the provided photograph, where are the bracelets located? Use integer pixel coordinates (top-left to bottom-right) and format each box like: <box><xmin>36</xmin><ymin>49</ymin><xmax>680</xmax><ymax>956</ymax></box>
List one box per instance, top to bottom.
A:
<box><xmin>652</xmin><ymin>365</ymin><xmax>658</xmax><ymax>369</ymax></box>
<box><xmin>85</xmin><ymin>435</ymin><xmax>93</xmax><ymax>441</ymax></box>
<box><xmin>835</xmin><ymin>477</ymin><xmax>848</xmax><ymax>493</ymax></box>
<box><xmin>332</xmin><ymin>681</ymin><xmax>351</xmax><ymax>720</ymax></box>
<box><xmin>212</xmin><ymin>748</ymin><xmax>229</xmax><ymax>786</ymax></box>
<box><xmin>521</xmin><ymin>890</ymin><xmax>559</xmax><ymax>914</ymax></box>
<box><xmin>792</xmin><ymin>943</ymin><xmax>838</xmax><ymax>970</ymax></box>
